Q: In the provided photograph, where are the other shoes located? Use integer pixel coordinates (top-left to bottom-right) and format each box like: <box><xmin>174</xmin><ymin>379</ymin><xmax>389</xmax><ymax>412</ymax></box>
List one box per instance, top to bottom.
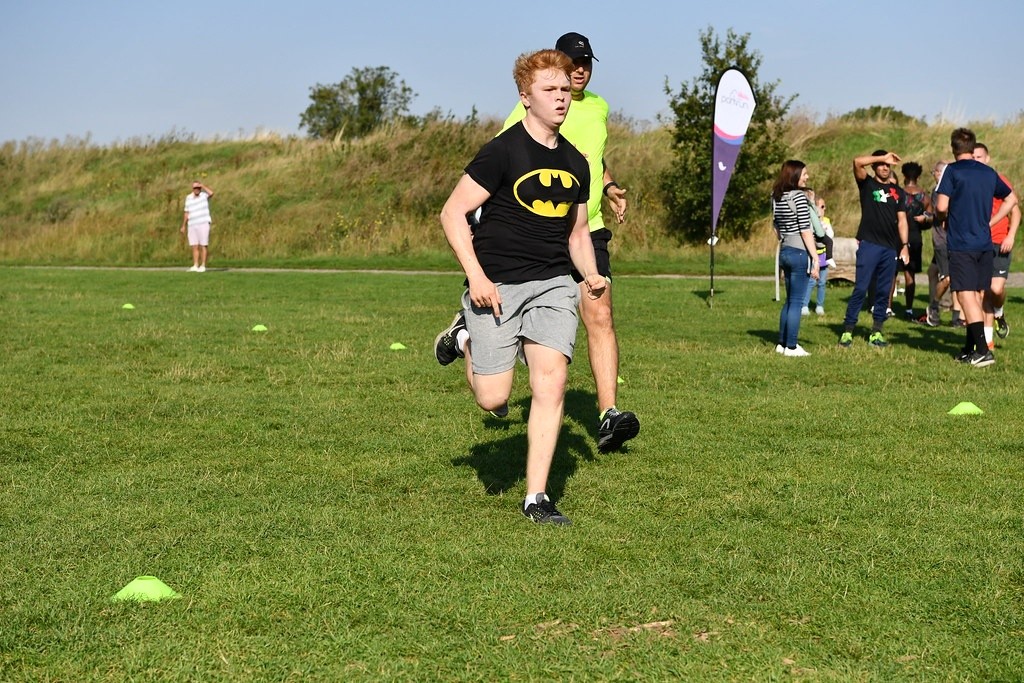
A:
<box><xmin>827</xmin><ymin>258</ymin><xmax>836</xmax><ymax>268</ymax></box>
<box><xmin>188</xmin><ymin>263</ymin><xmax>207</xmax><ymax>274</ymax></box>
<box><xmin>801</xmin><ymin>305</ymin><xmax>810</xmax><ymax>315</ymax></box>
<box><xmin>815</xmin><ymin>305</ymin><xmax>824</xmax><ymax>315</ymax></box>
<box><xmin>870</xmin><ymin>306</ymin><xmax>896</xmax><ymax>316</ymax></box>
<box><xmin>926</xmin><ymin>306</ymin><xmax>940</xmax><ymax>327</ymax></box>
<box><xmin>904</xmin><ymin>311</ymin><xmax>916</xmax><ymax>322</ymax></box>
<box><xmin>948</xmin><ymin>319</ymin><xmax>962</xmax><ymax>327</ymax></box>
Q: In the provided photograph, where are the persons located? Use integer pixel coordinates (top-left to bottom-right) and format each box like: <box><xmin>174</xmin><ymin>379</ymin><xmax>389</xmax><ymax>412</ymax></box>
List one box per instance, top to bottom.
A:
<box><xmin>475</xmin><ymin>32</ymin><xmax>646</xmax><ymax>456</ymax></box>
<box><xmin>433</xmin><ymin>48</ymin><xmax>608</xmax><ymax>526</ymax></box>
<box><xmin>937</xmin><ymin>128</ymin><xmax>1019</xmax><ymax>370</ymax></box>
<box><xmin>970</xmin><ymin>140</ymin><xmax>1022</xmax><ymax>350</ymax></box>
<box><xmin>839</xmin><ymin>149</ymin><xmax>912</xmax><ymax>347</ymax></box>
<box><xmin>769</xmin><ymin>160</ymin><xmax>821</xmax><ymax>358</ymax></box>
<box><xmin>886</xmin><ymin>160</ymin><xmax>934</xmax><ymax>319</ymax></box>
<box><xmin>914</xmin><ymin>160</ymin><xmax>953</xmax><ymax>327</ymax></box>
<box><xmin>178</xmin><ymin>178</ymin><xmax>215</xmax><ymax>273</ymax></box>
<box><xmin>803</xmin><ymin>187</ymin><xmax>838</xmax><ymax>268</ymax></box>
<box><xmin>801</xmin><ymin>191</ymin><xmax>835</xmax><ymax>317</ymax></box>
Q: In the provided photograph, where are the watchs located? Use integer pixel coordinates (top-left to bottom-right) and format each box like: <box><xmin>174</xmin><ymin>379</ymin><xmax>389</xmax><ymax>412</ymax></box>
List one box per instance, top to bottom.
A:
<box><xmin>901</xmin><ymin>242</ymin><xmax>911</xmax><ymax>248</ymax></box>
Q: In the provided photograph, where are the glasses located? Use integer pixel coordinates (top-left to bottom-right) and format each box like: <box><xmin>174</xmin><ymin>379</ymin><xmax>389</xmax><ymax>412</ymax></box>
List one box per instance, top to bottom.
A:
<box><xmin>816</xmin><ymin>205</ymin><xmax>825</xmax><ymax>210</ymax></box>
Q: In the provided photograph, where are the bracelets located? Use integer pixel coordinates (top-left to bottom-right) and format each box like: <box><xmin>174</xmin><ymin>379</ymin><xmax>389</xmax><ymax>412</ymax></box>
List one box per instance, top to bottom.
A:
<box><xmin>603</xmin><ymin>180</ymin><xmax>619</xmax><ymax>197</ymax></box>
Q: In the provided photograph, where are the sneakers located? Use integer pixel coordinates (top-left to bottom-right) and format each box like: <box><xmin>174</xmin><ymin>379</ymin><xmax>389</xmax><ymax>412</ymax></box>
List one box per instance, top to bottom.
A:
<box><xmin>435</xmin><ymin>309</ymin><xmax>469</xmax><ymax>366</ymax></box>
<box><xmin>993</xmin><ymin>315</ymin><xmax>1009</xmax><ymax>339</ymax></box>
<box><xmin>840</xmin><ymin>333</ymin><xmax>853</xmax><ymax>346</ymax></box>
<box><xmin>869</xmin><ymin>333</ymin><xmax>890</xmax><ymax>348</ymax></box>
<box><xmin>973</xmin><ymin>342</ymin><xmax>995</xmax><ymax>351</ymax></box>
<box><xmin>785</xmin><ymin>343</ymin><xmax>811</xmax><ymax>357</ymax></box>
<box><xmin>776</xmin><ymin>344</ymin><xmax>785</xmax><ymax>354</ymax></box>
<box><xmin>954</xmin><ymin>346</ymin><xmax>974</xmax><ymax>361</ymax></box>
<box><xmin>961</xmin><ymin>350</ymin><xmax>995</xmax><ymax>367</ymax></box>
<box><xmin>491</xmin><ymin>404</ymin><xmax>508</xmax><ymax>417</ymax></box>
<box><xmin>597</xmin><ymin>409</ymin><xmax>639</xmax><ymax>453</ymax></box>
<box><xmin>521</xmin><ymin>500</ymin><xmax>573</xmax><ymax>525</ymax></box>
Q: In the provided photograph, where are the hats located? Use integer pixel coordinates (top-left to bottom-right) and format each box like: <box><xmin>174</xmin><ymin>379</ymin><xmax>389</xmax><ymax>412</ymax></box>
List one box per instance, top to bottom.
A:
<box><xmin>555</xmin><ymin>32</ymin><xmax>598</xmax><ymax>62</ymax></box>
